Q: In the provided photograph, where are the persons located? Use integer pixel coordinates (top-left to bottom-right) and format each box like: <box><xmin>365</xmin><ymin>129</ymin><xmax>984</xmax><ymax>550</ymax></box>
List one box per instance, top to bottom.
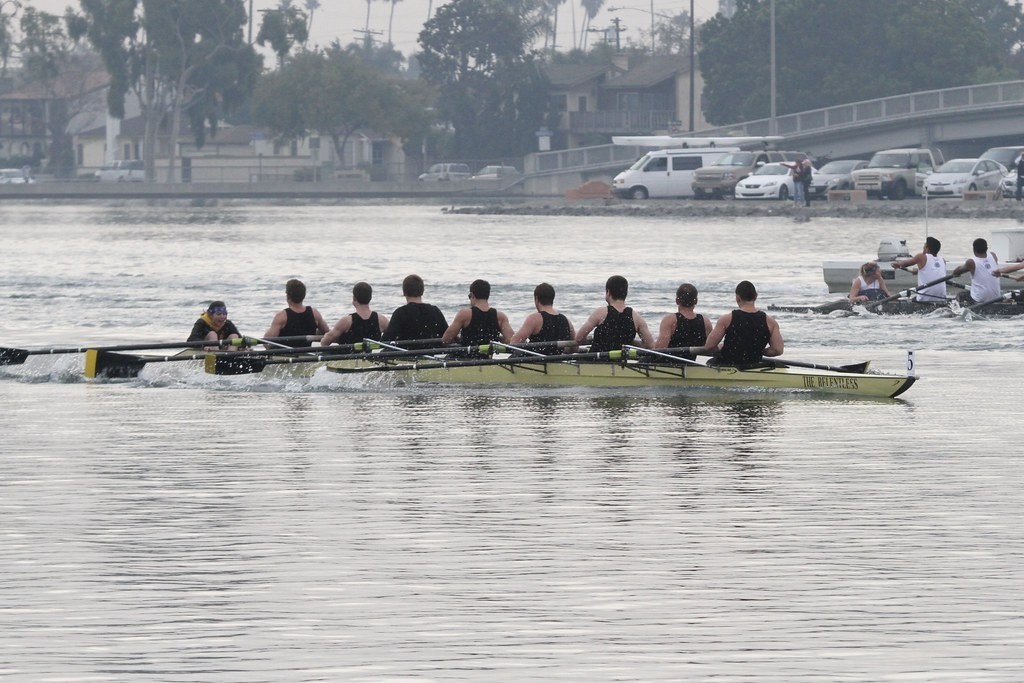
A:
<box><xmin>1014</xmin><ymin>152</ymin><xmax>1024</xmax><ymax>200</ymax></box>
<box><xmin>793</xmin><ymin>158</ymin><xmax>813</xmax><ymax>207</ymax></box>
<box><xmin>779</xmin><ymin>160</ymin><xmax>804</xmax><ymax>208</ymax></box>
<box><xmin>891</xmin><ymin>235</ymin><xmax>947</xmax><ymax>303</ymax></box>
<box><xmin>953</xmin><ymin>239</ymin><xmax>1000</xmax><ymax>303</ymax></box>
<box><xmin>991</xmin><ymin>253</ymin><xmax>1024</xmax><ymax>281</ymax></box>
<box><xmin>848</xmin><ymin>263</ymin><xmax>892</xmax><ymax>303</ymax></box>
<box><xmin>382</xmin><ymin>274</ymin><xmax>459</xmax><ymax>345</ymax></box>
<box><xmin>576</xmin><ymin>275</ymin><xmax>656</xmax><ymax>350</ymax></box>
<box><xmin>264</xmin><ymin>279</ymin><xmax>330</xmax><ymax>350</ymax></box>
<box><xmin>442</xmin><ymin>279</ymin><xmax>516</xmax><ymax>345</ymax></box>
<box><xmin>704</xmin><ymin>281</ymin><xmax>783</xmax><ymax>369</ymax></box>
<box><xmin>511</xmin><ymin>282</ymin><xmax>577</xmax><ymax>354</ymax></box>
<box><xmin>321</xmin><ymin>283</ymin><xmax>390</xmax><ymax>348</ymax></box>
<box><xmin>655</xmin><ymin>284</ymin><xmax>721</xmax><ymax>358</ymax></box>
<box><xmin>186</xmin><ymin>300</ymin><xmax>244</xmax><ymax>352</ymax></box>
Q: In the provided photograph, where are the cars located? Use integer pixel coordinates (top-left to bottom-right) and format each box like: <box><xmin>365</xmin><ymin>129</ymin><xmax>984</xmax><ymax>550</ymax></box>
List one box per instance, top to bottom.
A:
<box><xmin>978</xmin><ymin>145</ymin><xmax>1024</xmax><ymax>172</ymax></box>
<box><xmin>999</xmin><ymin>150</ymin><xmax>1024</xmax><ymax>197</ymax></box>
<box><xmin>914</xmin><ymin>158</ymin><xmax>1009</xmax><ymax>199</ymax></box>
<box><xmin>94</xmin><ymin>160</ymin><xmax>146</xmax><ymax>183</ymax></box>
<box><xmin>806</xmin><ymin>160</ymin><xmax>871</xmax><ymax>199</ymax></box>
<box><xmin>734</xmin><ymin>161</ymin><xmax>799</xmax><ymax>201</ymax></box>
<box><xmin>469</xmin><ymin>164</ymin><xmax>519</xmax><ymax>183</ymax></box>
<box><xmin>0</xmin><ymin>168</ymin><xmax>36</xmax><ymax>185</ymax></box>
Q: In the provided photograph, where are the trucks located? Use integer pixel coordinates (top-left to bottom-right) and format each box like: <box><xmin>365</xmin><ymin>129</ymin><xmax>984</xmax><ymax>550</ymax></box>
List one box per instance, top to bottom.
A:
<box><xmin>850</xmin><ymin>146</ymin><xmax>948</xmax><ymax>202</ymax></box>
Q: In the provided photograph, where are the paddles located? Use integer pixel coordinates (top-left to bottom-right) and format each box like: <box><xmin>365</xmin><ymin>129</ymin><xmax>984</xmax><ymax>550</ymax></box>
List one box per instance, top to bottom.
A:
<box><xmin>890</xmin><ymin>262</ymin><xmax>971</xmax><ymax>292</ymax></box>
<box><xmin>842</xmin><ymin>270</ymin><xmax>970</xmax><ymax>318</ymax></box>
<box><xmin>942</xmin><ymin>289</ymin><xmax>1024</xmax><ymax>317</ymax></box>
<box><xmin>0</xmin><ymin>334</ymin><xmax>330</xmax><ymax>367</ymax></box>
<box><xmin>84</xmin><ymin>336</ymin><xmax>444</xmax><ymax>380</ymax></box>
<box><xmin>204</xmin><ymin>337</ymin><xmax>594</xmax><ymax>377</ymax></box>
<box><xmin>326</xmin><ymin>349</ymin><xmax>652</xmax><ymax>374</ymax></box>
<box><xmin>722</xmin><ymin>349</ymin><xmax>872</xmax><ymax>375</ymax></box>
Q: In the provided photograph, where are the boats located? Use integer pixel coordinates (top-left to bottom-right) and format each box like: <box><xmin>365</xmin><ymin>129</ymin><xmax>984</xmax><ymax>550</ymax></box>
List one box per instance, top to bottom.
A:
<box><xmin>609</xmin><ymin>134</ymin><xmax>786</xmax><ymax>147</ymax></box>
<box><xmin>989</xmin><ymin>227</ymin><xmax>1024</xmax><ymax>263</ymax></box>
<box><xmin>822</xmin><ymin>235</ymin><xmax>1024</xmax><ymax>296</ymax></box>
<box><xmin>765</xmin><ymin>297</ymin><xmax>1024</xmax><ymax>317</ymax></box>
<box><xmin>81</xmin><ymin>346</ymin><xmax>875</xmax><ymax>384</ymax></box>
<box><xmin>195</xmin><ymin>350</ymin><xmax>921</xmax><ymax>399</ymax></box>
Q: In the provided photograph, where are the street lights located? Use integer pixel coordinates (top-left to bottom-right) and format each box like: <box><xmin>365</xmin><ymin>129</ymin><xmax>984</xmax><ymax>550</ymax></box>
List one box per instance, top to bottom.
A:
<box><xmin>605</xmin><ymin>5</ymin><xmax>672</xmax><ymax>54</ymax></box>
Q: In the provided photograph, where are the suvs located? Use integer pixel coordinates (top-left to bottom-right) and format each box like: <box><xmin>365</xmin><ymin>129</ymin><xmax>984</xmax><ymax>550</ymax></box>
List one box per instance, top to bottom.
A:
<box><xmin>690</xmin><ymin>149</ymin><xmax>816</xmax><ymax>201</ymax></box>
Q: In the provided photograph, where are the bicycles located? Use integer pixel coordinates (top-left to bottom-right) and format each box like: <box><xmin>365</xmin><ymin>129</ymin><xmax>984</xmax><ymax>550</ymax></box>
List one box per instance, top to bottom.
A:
<box><xmin>990</xmin><ymin>167</ymin><xmax>1018</xmax><ymax>202</ymax></box>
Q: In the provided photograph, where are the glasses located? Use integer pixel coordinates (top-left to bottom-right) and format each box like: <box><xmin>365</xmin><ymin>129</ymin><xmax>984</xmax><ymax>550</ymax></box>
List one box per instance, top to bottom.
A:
<box><xmin>468</xmin><ymin>292</ymin><xmax>472</xmax><ymax>299</ymax></box>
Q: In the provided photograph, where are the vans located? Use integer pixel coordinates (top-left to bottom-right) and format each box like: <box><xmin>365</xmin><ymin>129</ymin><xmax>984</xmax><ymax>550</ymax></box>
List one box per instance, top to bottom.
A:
<box><xmin>610</xmin><ymin>145</ymin><xmax>741</xmax><ymax>201</ymax></box>
<box><xmin>416</xmin><ymin>163</ymin><xmax>470</xmax><ymax>184</ymax></box>
